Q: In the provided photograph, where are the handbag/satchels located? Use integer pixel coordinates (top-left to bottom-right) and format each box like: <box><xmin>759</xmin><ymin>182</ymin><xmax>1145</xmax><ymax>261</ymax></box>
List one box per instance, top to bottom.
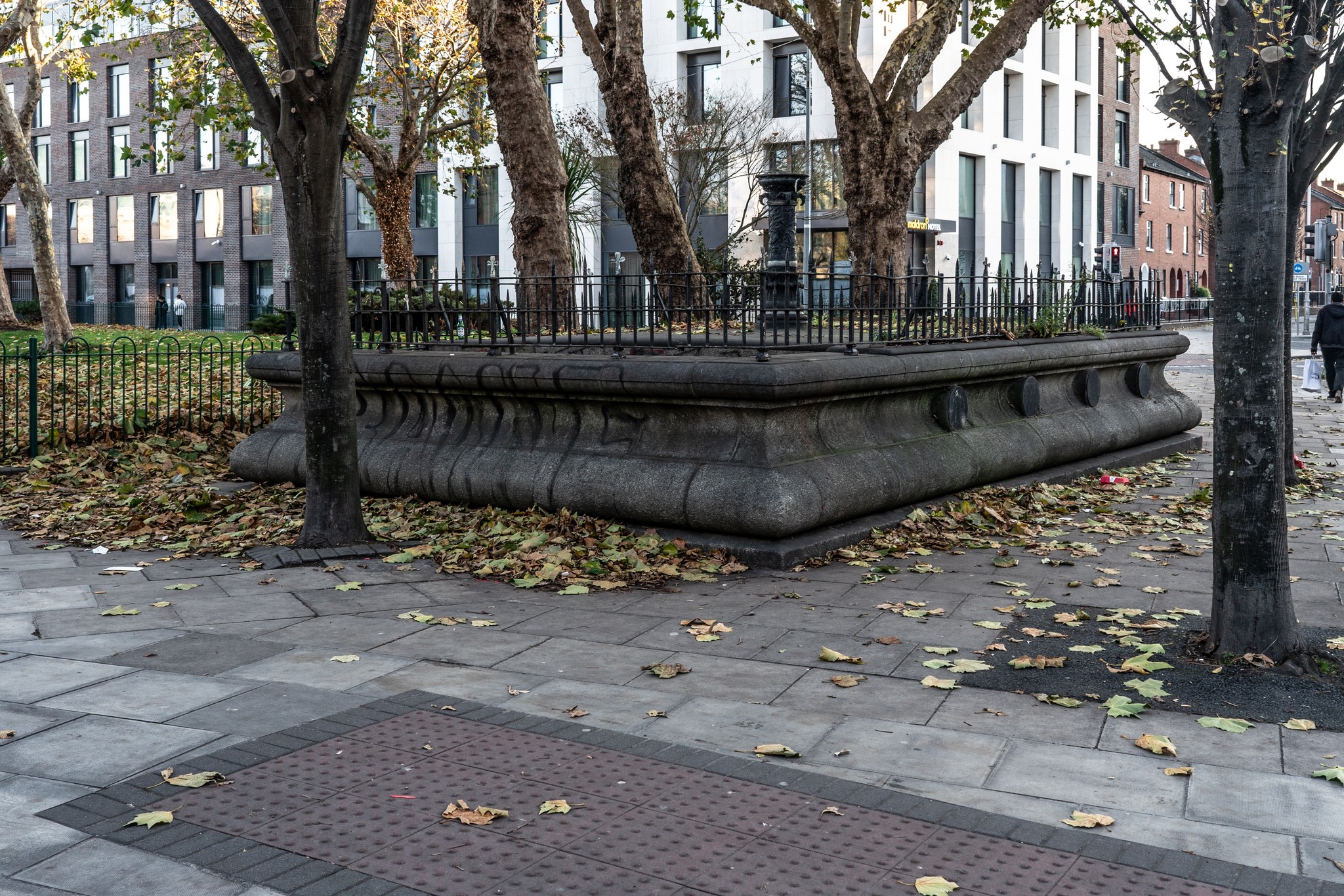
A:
<box><xmin>1300</xmin><ymin>353</ymin><xmax>1322</xmax><ymax>394</ymax></box>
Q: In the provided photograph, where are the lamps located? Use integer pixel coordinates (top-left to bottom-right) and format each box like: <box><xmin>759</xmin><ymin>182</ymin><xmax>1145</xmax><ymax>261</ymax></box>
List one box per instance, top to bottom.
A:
<box><xmin>1079</xmin><ymin>242</ymin><xmax>1084</xmax><ymax>248</ymax></box>
<box><xmin>946</xmin><ymin>254</ymin><xmax>951</xmax><ymax>260</ymax></box>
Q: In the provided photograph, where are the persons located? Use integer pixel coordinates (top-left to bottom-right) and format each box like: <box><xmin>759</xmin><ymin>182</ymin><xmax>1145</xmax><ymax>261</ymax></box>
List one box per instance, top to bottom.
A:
<box><xmin>1299</xmin><ymin>284</ymin><xmax>1305</xmax><ymax>301</ymax></box>
<box><xmin>1311</xmin><ymin>292</ymin><xmax>1344</xmax><ymax>403</ymax></box>
<box><xmin>155</xmin><ymin>294</ymin><xmax>168</xmax><ymax>329</ymax></box>
<box><xmin>174</xmin><ymin>294</ymin><xmax>187</xmax><ymax>331</ymax></box>
<box><xmin>1124</xmin><ymin>295</ymin><xmax>1139</xmax><ymax>330</ymax></box>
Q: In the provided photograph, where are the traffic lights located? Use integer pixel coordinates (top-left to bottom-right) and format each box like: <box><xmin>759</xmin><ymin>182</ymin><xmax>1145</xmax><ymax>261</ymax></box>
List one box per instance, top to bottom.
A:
<box><xmin>1304</xmin><ymin>223</ymin><xmax>1325</xmax><ymax>262</ymax></box>
<box><xmin>1094</xmin><ymin>246</ymin><xmax>1106</xmax><ymax>273</ymax></box>
<box><xmin>1109</xmin><ymin>246</ymin><xmax>1120</xmax><ymax>275</ymax></box>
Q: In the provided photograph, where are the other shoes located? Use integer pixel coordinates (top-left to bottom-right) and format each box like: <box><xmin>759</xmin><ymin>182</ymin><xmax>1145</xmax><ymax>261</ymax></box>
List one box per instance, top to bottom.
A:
<box><xmin>1335</xmin><ymin>391</ymin><xmax>1343</xmax><ymax>403</ymax></box>
<box><xmin>1327</xmin><ymin>395</ymin><xmax>1336</xmax><ymax>400</ymax></box>
